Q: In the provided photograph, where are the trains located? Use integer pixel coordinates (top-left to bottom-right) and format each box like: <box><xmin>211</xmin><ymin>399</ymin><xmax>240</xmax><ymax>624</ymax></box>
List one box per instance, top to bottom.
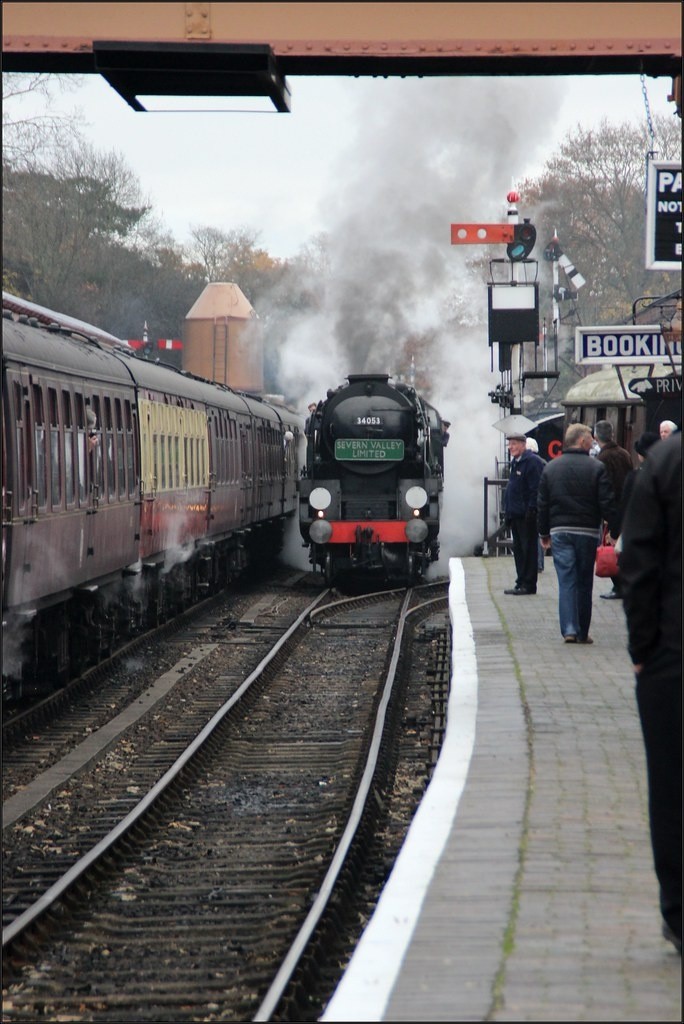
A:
<box><xmin>1</xmin><ymin>291</ymin><xmax>307</xmax><ymax>708</ymax></box>
<box><xmin>298</xmin><ymin>370</ymin><xmax>451</xmax><ymax>589</ymax></box>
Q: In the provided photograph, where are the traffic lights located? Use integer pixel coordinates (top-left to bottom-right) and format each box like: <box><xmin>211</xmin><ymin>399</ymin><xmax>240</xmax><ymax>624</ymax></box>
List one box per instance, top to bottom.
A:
<box><xmin>507</xmin><ymin>224</ymin><xmax>537</xmax><ymax>261</ymax></box>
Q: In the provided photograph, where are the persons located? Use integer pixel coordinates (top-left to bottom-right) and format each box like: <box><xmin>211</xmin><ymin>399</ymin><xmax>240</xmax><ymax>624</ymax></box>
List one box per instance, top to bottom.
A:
<box><xmin>304</xmin><ymin>403</ymin><xmax>317</xmax><ymax>435</ymax></box>
<box><xmin>85</xmin><ymin>405</ymin><xmax>98</xmax><ymax>454</ymax></box>
<box><xmin>503</xmin><ymin>420</ymin><xmax>676</xmax><ymax>644</ymax></box>
<box><xmin>442</xmin><ymin>421</ymin><xmax>451</xmax><ymax>448</ymax></box>
<box><xmin>285</xmin><ymin>431</ymin><xmax>293</xmax><ymax>448</ymax></box>
<box><xmin>616</xmin><ymin>433</ymin><xmax>683</xmax><ymax>957</ymax></box>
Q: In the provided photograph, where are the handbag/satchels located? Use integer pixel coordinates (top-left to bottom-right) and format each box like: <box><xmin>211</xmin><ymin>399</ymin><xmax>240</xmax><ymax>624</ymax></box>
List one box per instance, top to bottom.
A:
<box><xmin>596</xmin><ymin>525</ymin><xmax>620</xmax><ymax>576</ymax></box>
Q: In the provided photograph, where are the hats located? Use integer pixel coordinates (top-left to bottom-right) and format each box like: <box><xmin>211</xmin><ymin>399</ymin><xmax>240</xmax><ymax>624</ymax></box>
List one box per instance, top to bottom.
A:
<box><xmin>506</xmin><ymin>433</ymin><xmax>526</xmax><ymax>442</ymax></box>
<box><xmin>634</xmin><ymin>433</ymin><xmax>658</xmax><ymax>456</ymax></box>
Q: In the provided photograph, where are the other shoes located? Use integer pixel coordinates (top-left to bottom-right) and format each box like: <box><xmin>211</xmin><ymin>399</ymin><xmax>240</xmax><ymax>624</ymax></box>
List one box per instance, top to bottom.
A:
<box><xmin>538</xmin><ymin>570</ymin><xmax>542</xmax><ymax>573</ymax></box>
<box><xmin>504</xmin><ymin>586</ymin><xmax>536</xmax><ymax>594</ymax></box>
<box><xmin>599</xmin><ymin>591</ymin><xmax>622</xmax><ymax>599</ymax></box>
<box><xmin>564</xmin><ymin>636</ymin><xmax>576</xmax><ymax>643</ymax></box>
<box><xmin>576</xmin><ymin>636</ymin><xmax>593</xmax><ymax>643</ymax></box>
<box><xmin>661</xmin><ymin>920</ymin><xmax>682</xmax><ymax>953</ymax></box>
